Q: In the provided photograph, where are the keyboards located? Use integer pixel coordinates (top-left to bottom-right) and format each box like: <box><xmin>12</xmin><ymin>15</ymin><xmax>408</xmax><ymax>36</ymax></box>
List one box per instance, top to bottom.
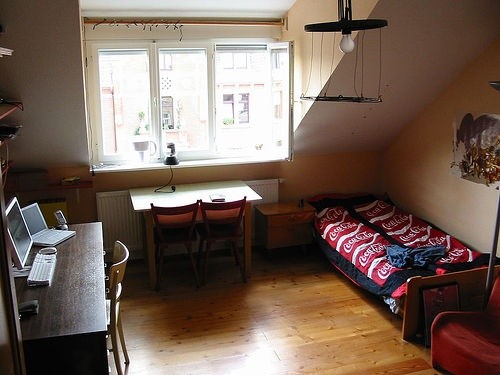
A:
<box><xmin>26</xmin><ymin>252</ymin><xmax>56</xmax><ymax>287</ymax></box>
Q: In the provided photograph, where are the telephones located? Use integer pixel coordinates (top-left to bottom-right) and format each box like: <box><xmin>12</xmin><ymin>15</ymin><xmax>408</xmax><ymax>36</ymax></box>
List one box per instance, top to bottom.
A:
<box><xmin>54</xmin><ymin>210</ymin><xmax>68</xmax><ymax>230</ymax></box>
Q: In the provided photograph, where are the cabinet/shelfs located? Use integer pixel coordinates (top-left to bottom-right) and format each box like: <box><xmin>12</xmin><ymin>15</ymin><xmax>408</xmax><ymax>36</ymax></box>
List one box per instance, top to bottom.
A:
<box><xmin>0</xmin><ymin>103</ymin><xmax>24</xmax><ymax>177</ymax></box>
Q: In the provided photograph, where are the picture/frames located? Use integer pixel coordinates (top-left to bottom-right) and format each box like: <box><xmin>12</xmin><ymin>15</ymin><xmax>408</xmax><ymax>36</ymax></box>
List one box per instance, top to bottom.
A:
<box><xmin>419</xmin><ymin>282</ymin><xmax>461</xmax><ymax>348</ymax></box>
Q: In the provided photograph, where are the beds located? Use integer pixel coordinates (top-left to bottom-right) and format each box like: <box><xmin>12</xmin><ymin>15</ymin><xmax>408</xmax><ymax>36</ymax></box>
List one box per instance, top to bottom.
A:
<box><xmin>306</xmin><ymin>191</ymin><xmax>500</xmax><ymax>339</ymax></box>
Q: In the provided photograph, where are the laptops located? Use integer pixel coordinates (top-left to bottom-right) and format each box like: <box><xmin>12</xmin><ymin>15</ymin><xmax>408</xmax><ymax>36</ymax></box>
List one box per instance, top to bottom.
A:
<box><xmin>21</xmin><ymin>202</ymin><xmax>76</xmax><ymax>247</ymax></box>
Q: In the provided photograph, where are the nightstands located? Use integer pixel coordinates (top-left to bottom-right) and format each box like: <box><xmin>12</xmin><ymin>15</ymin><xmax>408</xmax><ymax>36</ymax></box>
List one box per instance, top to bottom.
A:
<box><xmin>255</xmin><ymin>200</ymin><xmax>317</xmax><ymax>262</ymax></box>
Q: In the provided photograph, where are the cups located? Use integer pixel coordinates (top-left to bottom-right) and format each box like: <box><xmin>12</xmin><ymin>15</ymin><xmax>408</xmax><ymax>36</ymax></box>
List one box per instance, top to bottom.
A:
<box><xmin>299</xmin><ymin>199</ymin><xmax>304</xmax><ymax>208</ymax></box>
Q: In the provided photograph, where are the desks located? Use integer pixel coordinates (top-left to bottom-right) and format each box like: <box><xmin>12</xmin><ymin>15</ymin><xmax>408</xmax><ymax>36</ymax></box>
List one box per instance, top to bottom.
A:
<box><xmin>129</xmin><ymin>178</ymin><xmax>263</xmax><ymax>291</ymax></box>
<box><xmin>16</xmin><ymin>222</ymin><xmax>109</xmax><ymax>375</ymax></box>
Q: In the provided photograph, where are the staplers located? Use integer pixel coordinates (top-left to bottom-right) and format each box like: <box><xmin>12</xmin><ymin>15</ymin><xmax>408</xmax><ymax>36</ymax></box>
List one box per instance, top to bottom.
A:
<box><xmin>17</xmin><ymin>299</ymin><xmax>40</xmax><ymax>316</ymax></box>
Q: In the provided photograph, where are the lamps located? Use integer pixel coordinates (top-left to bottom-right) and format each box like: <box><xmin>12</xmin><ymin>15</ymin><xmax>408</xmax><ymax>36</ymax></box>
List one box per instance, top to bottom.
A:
<box><xmin>300</xmin><ymin>0</ymin><xmax>389</xmax><ymax>103</ymax></box>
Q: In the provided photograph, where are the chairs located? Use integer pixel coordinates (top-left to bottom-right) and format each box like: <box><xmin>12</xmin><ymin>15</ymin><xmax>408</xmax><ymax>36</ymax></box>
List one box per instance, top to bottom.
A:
<box><xmin>197</xmin><ymin>196</ymin><xmax>247</xmax><ymax>285</ymax></box>
<box><xmin>149</xmin><ymin>200</ymin><xmax>199</xmax><ymax>290</ymax></box>
<box><xmin>106</xmin><ymin>240</ymin><xmax>130</xmax><ymax>375</ymax></box>
<box><xmin>430</xmin><ymin>277</ymin><xmax>500</xmax><ymax>375</ymax></box>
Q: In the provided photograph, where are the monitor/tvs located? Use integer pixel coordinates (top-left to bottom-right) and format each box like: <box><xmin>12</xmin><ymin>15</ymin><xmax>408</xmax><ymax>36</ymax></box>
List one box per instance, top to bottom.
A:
<box><xmin>5</xmin><ymin>196</ymin><xmax>34</xmax><ymax>278</ymax></box>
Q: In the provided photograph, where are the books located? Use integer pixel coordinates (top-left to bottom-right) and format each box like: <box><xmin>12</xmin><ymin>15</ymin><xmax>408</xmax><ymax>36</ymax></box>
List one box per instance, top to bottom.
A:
<box><xmin>209</xmin><ymin>194</ymin><xmax>225</xmax><ymax>202</ymax></box>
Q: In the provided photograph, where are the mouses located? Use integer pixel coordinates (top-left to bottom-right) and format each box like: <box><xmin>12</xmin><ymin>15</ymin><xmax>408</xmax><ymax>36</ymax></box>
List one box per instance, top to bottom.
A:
<box><xmin>39</xmin><ymin>247</ymin><xmax>57</xmax><ymax>254</ymax></box>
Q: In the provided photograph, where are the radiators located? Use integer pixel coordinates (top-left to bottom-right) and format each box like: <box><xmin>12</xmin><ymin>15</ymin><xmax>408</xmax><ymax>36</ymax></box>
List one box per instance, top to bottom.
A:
<box><xmin>95</xmin><ymin>178</ymin><xmax>280</xmax><ymax>262</ymax></box>
